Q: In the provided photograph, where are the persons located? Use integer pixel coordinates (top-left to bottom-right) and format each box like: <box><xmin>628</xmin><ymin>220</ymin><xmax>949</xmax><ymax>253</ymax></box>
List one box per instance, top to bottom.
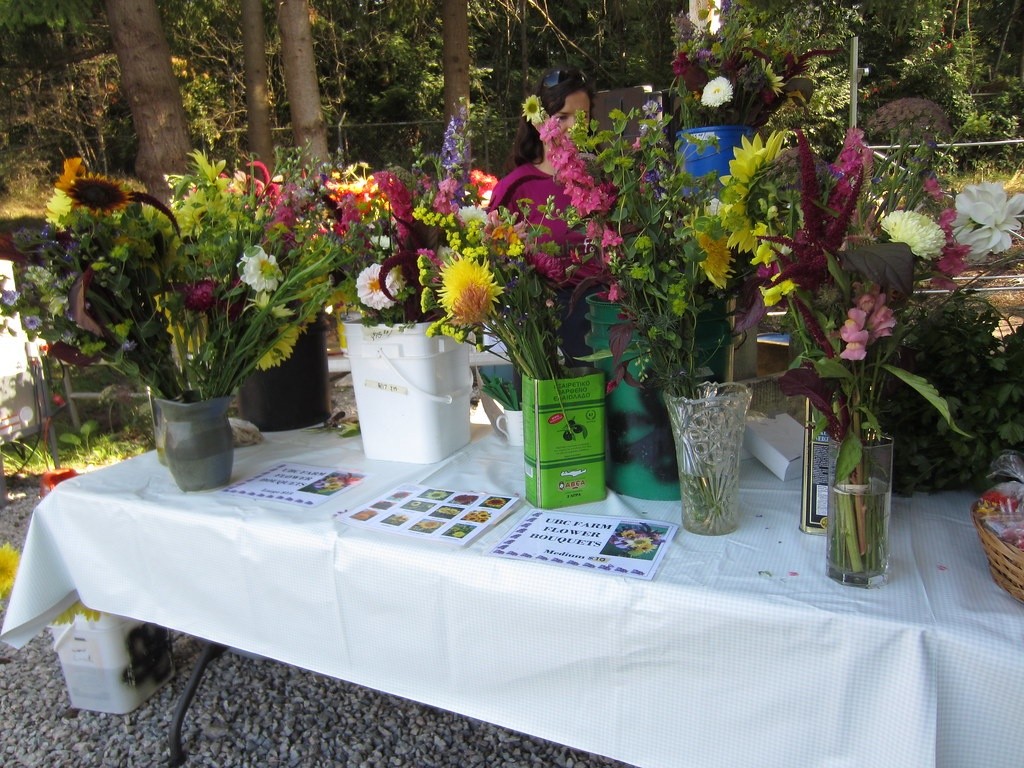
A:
<box><xmin>487</xmin><ymin>67</ymin><xmax>630</xmax><ymax>412</ymax></box>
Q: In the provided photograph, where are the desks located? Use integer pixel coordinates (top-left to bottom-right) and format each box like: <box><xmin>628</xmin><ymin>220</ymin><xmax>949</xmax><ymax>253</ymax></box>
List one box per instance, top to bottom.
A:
<box><xmin>1</xmin><ymin>425</ymin><xmax>1024</xmax><ymax>766</ymax></box>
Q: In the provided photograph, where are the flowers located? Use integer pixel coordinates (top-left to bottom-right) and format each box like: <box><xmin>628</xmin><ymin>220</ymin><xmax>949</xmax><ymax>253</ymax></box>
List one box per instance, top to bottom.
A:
<box><xmin>666</xmin><ymin>3</ymin><xmax>777</xmax><ymax>105</ymax></box>
<box><xmin>47</xmin><ymin>113</ymin><xmax>1012</xmax><ymax>414</ymax></box>
<box><xmin>0</xmin><ymin>544</ymin><xmax>100</xmax><ymax>625</ymax></box>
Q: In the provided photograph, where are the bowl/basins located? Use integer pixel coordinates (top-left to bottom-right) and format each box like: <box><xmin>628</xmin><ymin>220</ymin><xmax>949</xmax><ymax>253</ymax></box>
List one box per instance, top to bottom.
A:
<box><xmin>41</xmin><ymin>469</ymin><xmax>77</xmax><ymax>497</ymax></box>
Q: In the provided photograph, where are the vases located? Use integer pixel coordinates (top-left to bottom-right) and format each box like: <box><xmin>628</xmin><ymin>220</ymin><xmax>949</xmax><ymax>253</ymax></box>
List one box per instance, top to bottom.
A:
<box><xmin>673</xmin><ymin>124</ymin><xmax>754</xmax><ymax>192</ymax></box>
<box><xmin>584</xmin><ymin>291</ymin><xmax>680</xmax><ymax>500</ymax></box>
<box><xmin>235</xmin><ymin>311</ymin><xmax>330</xmax><ymax>432</ymax></box>
<box><xmin>348</xmin><ymin>316</ymin><xmax>471</xmax><ymax>464</ymax></box>
<box><xmin>521</xmin><ymin>368</ymin><xmax>605</xmax><ymax>509</ymax></box>
<box><xmin>664</xmin><ymin>381</ymin><xmax>753</xmax><ymax>536</ymax></box>
<box><xmin>157</xmin><ymin>391</ymin><xmax>234</xmax><ymax>492</ymax></box>
<box><xmin>830</xmin><ymin>438</ymin><xmax>893</xmax><ymax>588</ymax></box>
<box><xmin>49</xmin><ymin>601</ymin><xmax>178</xmax><ymax>712</ymax></box>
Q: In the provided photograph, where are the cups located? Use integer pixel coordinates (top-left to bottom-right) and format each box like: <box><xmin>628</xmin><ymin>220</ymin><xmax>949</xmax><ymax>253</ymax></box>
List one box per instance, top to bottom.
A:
<box><xmin>661</xmin><ymin>382</ymin><xmax>752</xmax><ymax>537</ymax></box>
<box><xmin>495</xmin><ymin>402</ymin><xmax>523</xmax><ymax>446</ymax></box>
<box><xmin>824</xmin><ymin>434</ymin><xmax>894</xmax><ymax>591</ymax></box>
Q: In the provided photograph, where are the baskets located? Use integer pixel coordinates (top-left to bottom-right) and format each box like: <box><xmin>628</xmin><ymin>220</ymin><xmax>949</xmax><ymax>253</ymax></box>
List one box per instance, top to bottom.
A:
<box><xmin>972</xmin><ymin>497</ymin><xmax>1024</xmax><ymax>602</ymax></box>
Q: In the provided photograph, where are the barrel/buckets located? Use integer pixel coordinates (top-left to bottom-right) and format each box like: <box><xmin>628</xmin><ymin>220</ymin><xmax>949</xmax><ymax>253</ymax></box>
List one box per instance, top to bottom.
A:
<box><xmin>676</xmin><ymin>126</ymin><xmax>756</xmax><ymax>200</ymax></box>
<box><xmin>586</xmin><ymin>289</ymin><xmax>728</xmax><ymax>500</ymax></box>
<box><xmin>238</xmin><ymin>308</ymin><xmax>331</xmax><ymax>434</ymax></box>
<box><xmin>344</xmin><ymin>310</ymin><xmax>471</xmax><ymax>464</ymax></box>
<box><xmin>48</xmin><ymin>601</ymin><xmax>175</xmax><ymax>714</ymax></box>
